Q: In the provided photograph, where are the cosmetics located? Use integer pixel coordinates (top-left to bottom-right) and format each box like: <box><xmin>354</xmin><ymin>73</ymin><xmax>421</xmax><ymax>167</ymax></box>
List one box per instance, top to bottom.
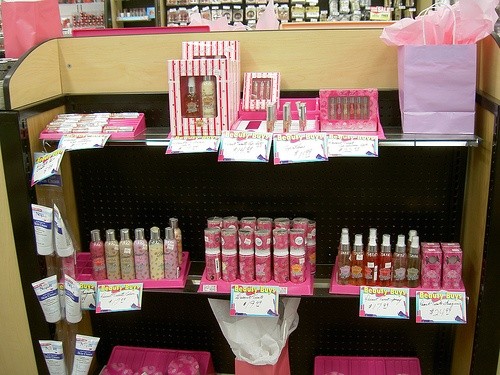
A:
<box><xmin>33</xmin><ymin>41</ymin><xmax>462</xmax><ymax>375</ymax></box>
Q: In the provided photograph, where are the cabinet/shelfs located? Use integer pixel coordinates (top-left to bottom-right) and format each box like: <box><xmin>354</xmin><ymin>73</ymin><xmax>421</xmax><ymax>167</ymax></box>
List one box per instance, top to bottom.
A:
<box><xmin>0</xmin><ymin>21</ymin><xmax>499</xmax><ymax>375</ymax></box>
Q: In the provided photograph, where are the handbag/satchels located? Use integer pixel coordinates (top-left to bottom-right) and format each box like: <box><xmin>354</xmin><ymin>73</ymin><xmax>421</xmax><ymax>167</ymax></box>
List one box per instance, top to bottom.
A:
<box><xmin>396</xmin><ymin>2</ymin><xmax>476</xmax><ymax>136</ymax></box>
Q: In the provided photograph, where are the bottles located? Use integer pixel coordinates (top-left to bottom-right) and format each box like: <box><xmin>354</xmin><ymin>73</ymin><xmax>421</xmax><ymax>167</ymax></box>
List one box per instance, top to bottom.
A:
<box><xmin>90</xmin><ymin>217</ymin><xmax>182</xmax><ymax>281</ymax></box>
<box><xmin>336</xmin><ymin>226</ymin><xmax>420</xmax><ymax>289</ymax></box>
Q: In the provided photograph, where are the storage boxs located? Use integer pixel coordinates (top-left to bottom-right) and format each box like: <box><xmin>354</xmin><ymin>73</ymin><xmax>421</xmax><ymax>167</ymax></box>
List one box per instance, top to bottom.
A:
<box><xmin>167</xmin><ymin>39</ymin><xmax>280</xmax><ymax>139</ymax></box>
<box><xmin>318</xmin><ymin>88</ymin><xmax>378</xmax><ymax>131</ymax></box>
